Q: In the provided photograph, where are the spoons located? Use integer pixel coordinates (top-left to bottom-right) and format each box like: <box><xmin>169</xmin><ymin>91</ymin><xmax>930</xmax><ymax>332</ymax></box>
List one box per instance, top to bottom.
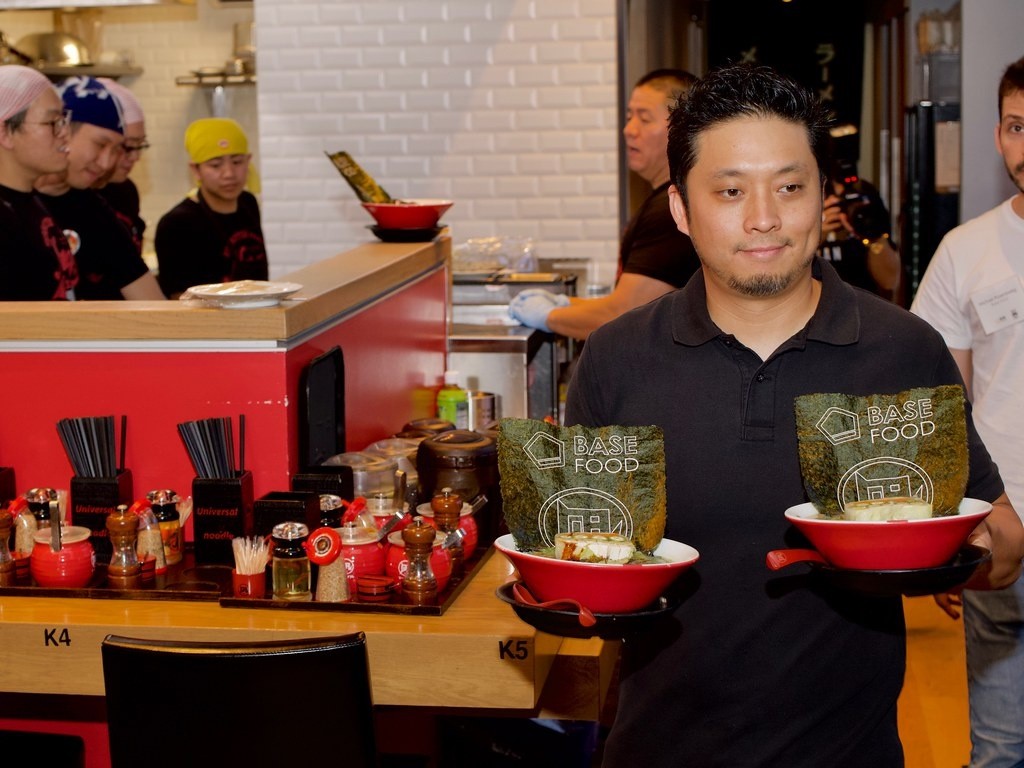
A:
<box><xmin>764</xmin><ymin>550</ymin><xmax>826</xmax><ymax>570</ymax></box>
<box><xmin>512</xmin><ymin>581</ymin><xmax>604</xmax><ymax>632</ymax></box>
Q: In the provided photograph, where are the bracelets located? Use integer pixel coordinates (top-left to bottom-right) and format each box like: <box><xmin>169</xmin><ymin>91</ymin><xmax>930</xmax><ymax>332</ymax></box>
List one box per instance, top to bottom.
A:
<box><xmin>865</xmin><ymin>241</ymin><xmax>888</xmax><ymax>254</ymax></box>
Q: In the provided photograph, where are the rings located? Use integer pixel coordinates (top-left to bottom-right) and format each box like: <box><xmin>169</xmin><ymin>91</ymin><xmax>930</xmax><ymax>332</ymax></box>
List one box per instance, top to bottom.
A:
<box><xmin>822</xmin><ymin>212</ymin><xmax>826</xmax><ymax>222</ymax></box>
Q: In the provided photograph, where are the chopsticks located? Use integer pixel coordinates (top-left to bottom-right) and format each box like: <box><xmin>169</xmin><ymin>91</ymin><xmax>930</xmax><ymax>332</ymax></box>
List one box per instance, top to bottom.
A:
<box><xmin>55</xmin><ymin>414</ymin><xmax>128</xmax><ymax>481</ymax></box>
<box><xmin>177</xmin><ymin>414</ymin><xmax>245</xmax><ymax>479</ymax></box>
<box><xmin>231</xmin><ymin>536</ymin><xmax>271</xmax><ymax>573</ymax></box>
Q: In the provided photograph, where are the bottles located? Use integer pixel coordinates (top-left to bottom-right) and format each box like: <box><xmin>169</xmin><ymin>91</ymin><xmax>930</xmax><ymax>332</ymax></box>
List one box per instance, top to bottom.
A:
<box><xmin>435</xmin><ymin>367</ymin><xmax>468</xmax><ymax>431</ymax></box>
<box><xmin>235</xmin><ymin>461</ymin><xmax>477</xmax><ymax>608</ymax></box>
<box><xmin>1</xmin><ymin>488</ymin><xmax>60</xmax><ymax>571</ymax></box>
<box><xmin>106</xmin><ymin>489</ymin><xmax>183</xmax><ymax>588</ymax></box>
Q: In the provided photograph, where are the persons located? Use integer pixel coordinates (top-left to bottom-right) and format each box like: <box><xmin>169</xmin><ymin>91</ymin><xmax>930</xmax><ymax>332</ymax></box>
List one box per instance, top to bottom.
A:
<box><xmin>816</xmin><ymin>53</ymin><xmax>1024</xmax><ymax>768</ymax></box>
<box><xmin>0</xmin><ymin>65</ymin><xmax>167</xmax><ymax>301</ymax></box>
<box><xmin>564</xmin><ymin>65</ymin><xmax>1024</xmax><ymax>768</ymax></box>
<box><xmin>509</xmin><ymin>66</ymin><xmax>705</xmax><ymax>341</ymax></box>
<box><xmin>154</xmin><ymin>118</ymin><xmax>269</xmax><ymax>300</ymax></box>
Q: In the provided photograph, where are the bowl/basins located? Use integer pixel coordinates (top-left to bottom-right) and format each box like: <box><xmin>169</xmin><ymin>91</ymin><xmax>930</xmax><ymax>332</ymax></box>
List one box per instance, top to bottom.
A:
<box><xmin>360</xmin><ymin>198</ymin><xmax>454</xmax><ymax>229</ymax></box>
<box><xmin>783</xmin><ymin>497</ymin><xmax>998</xmax><ymax>573</ymax></box>
<box><xmin>487</xmin><ymin>532</ymin><xmax>702</xmax><ymax>613</ymax></box>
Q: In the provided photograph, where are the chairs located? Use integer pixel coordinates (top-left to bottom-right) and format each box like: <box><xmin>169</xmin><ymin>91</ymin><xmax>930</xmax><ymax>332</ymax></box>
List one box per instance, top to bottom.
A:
<box><xmin>99</xmin><ymin>629</ymin><xmax>381</xmax><ymax>768</ymax></box>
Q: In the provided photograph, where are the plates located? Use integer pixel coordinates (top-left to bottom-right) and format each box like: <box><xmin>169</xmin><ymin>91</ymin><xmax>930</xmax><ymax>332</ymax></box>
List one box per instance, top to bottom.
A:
<box><xmin>365</xmin><ymin>224</ymin><xmax>449</xmax><ymax>243</ymax></box>
<box><xmin>187</xmin><ymin>281</ymin><xmax>303</xmax><ymax>309</ymax></box>
<box><xmin>783</xmin><ymin>524</ymin><xmax>992</xmax><ymax>596</ymax></box>
<box><xmin>495</xmin><ymin>579</ymin><xmax>671</xmax><ymax>640</ymax></box>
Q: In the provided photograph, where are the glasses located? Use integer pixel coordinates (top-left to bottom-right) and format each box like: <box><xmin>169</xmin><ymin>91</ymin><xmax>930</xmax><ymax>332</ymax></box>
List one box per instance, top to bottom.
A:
<box><xmin>8</xmin><ymin>108</ymin><xmax>73</xmax><ymax>137</ymax></box>
<box><xmin>122</xmin><ymin>139</ymin><xmax>152</xmax><ymax>156</ymax></box>
